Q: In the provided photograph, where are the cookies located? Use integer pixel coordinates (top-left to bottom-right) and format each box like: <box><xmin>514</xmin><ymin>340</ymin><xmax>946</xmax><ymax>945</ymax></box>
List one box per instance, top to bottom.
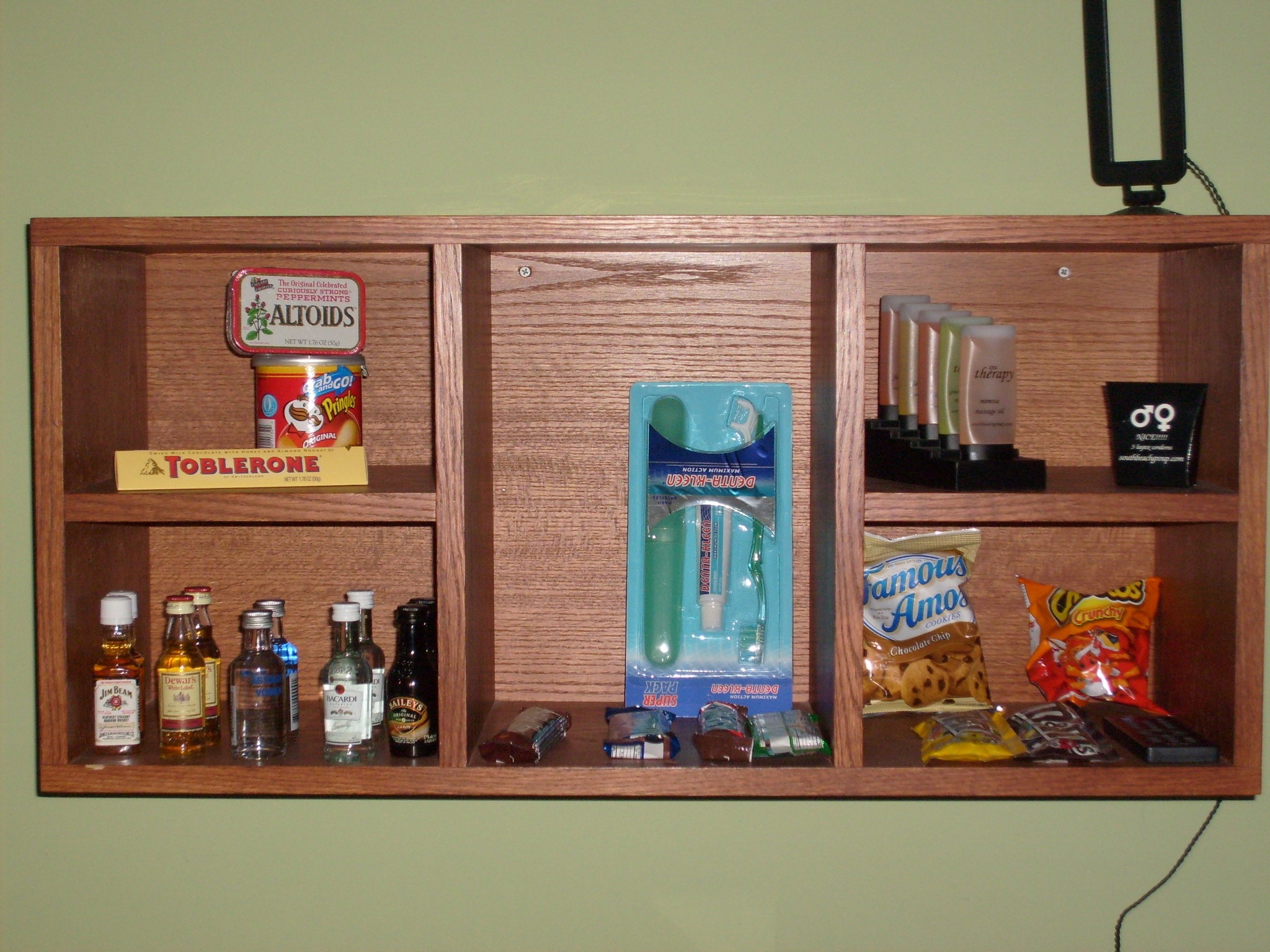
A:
<box><xmin>863</xmin><ymin>634</ymin><xmax>992</xmax><ymax>708</ymax></box>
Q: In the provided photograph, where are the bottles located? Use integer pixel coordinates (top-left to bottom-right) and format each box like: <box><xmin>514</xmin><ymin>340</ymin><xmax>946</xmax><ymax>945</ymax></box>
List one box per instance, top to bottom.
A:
<box><xmin>185</xmin><ymin>586</ymin><xmax>220</xmax><ymax>749</ymax></box>
<box><xmin>344</xmin><ymin>590</ymin><xmax>385</xmax><ymax>742</ymax></box>
<box><xmin>104</xmin><ymin>591</ymin><xmax>147</xmax><ymax>749</ymax></box>
<box><xmin>155</xmin><ymin>595</ymin><xmax>208</xmax><ymax>758</ymax></box>
<box><xmin>89</xmin><ymin>596</ymin><xmax>143</xmax><ymax>758</ymax></box>
<box><xmin>405</xmin><ymin>598</ymin><xmax>438</xmax><ymax>754</ymax></box>
<box><xmin>255</xmin><ymin>600</ymin><xmax>299</xmax><ymax>741</ymax></box>
<box><xmin>321</xmin><ymin>603</ymin><xmax>373</xmax><ymax>765</ymax></box>
<box><xmin>383</xmin><ymin>605</ymin><xmax>438</xmax><ymax>758</ymax></box>
<box><xmin>228</xmin><ymin>609</ymin><xmax>286</xmax><ymax>761</ymax></box>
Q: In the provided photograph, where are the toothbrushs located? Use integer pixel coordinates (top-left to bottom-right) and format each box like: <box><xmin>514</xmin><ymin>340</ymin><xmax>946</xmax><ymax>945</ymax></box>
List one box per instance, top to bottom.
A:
<box><xmin>736</xmin><ymin>413</ymin><xmax>768</xmax><ymax>665</ymax></box>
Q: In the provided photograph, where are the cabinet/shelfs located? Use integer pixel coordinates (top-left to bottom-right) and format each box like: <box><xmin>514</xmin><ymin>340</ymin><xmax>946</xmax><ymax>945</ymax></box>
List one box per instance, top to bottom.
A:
<box><xmin>30</xmin><ymin>215</ymin><xmax>1270</xmax><ymax>803</ymax></box>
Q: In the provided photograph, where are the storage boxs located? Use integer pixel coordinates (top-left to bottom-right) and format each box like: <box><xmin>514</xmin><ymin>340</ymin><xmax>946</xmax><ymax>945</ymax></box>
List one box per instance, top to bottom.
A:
<box><xmin>224</xmin><ymin>266</ymin><xmax>366</xmax><ymax>357</ymax></box>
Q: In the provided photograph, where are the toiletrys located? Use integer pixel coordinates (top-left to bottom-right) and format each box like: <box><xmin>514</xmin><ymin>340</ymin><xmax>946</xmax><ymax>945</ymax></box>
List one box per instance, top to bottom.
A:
<box><xmin>875</xmin><ymin>294</ymin><xmax>1018</xmax><ymax>464</ymax></box>
<box><xmin>1103</xmin><ymin>378</ymin><xmax>1210</xmax><ymax>488</ymax></box>
<box><xmin>696</xmin><ymin>505</ymin><xmax>733</xmax><ymax>633</ymax></box>
